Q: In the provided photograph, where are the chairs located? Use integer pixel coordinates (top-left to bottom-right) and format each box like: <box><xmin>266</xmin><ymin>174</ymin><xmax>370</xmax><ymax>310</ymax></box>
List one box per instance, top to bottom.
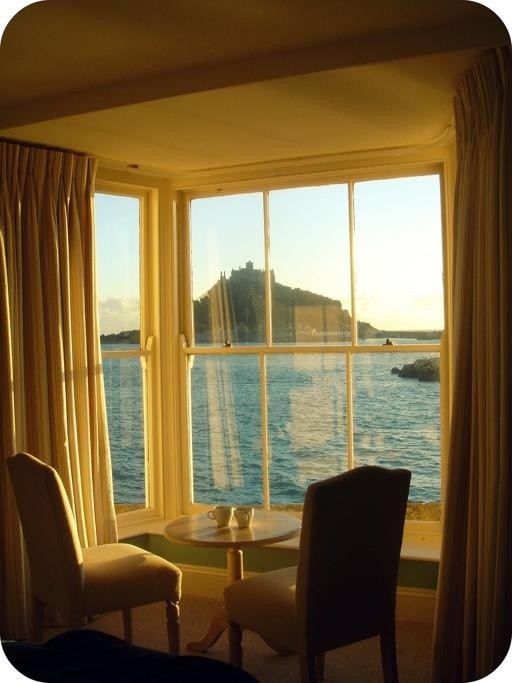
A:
<box><xmin>4</xmin><ymin>452</ymin><xmax>182</xmax><ymax>656</ymax></box>
<box><xmin>223</xmin><ymin>466</ymin><xmax>412</xmax><ymax>682</ymax></box>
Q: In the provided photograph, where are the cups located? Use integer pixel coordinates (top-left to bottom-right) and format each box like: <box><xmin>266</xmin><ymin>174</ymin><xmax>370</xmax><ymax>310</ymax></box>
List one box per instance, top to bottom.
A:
<box><xmin>207</xmin><ymin>505</ymin><xmax>254</xmax><ymax>529</ymax></box>
